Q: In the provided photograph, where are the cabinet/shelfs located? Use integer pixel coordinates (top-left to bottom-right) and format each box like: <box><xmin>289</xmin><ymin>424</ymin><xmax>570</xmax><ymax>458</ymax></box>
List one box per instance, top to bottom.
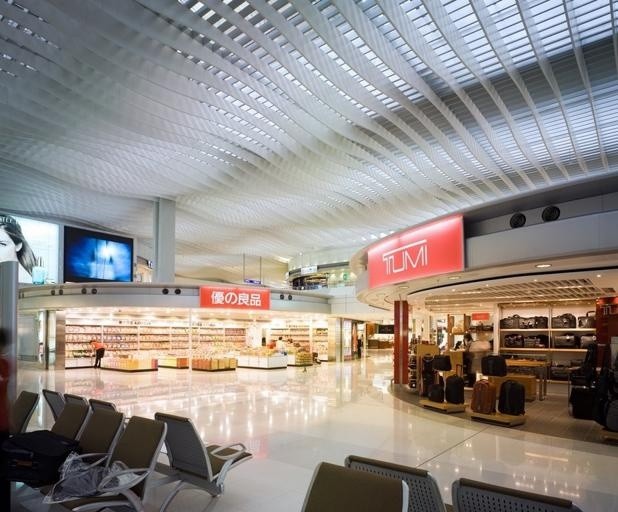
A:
<box><xmin>402</xmin><ymin>297</ymin><xmax>618</xmax><ymax>424</ymax></box>
<box><xmin>64</xmin><ymin>324</ymin><xmax>330</xmax><ymax>370</ymax></box>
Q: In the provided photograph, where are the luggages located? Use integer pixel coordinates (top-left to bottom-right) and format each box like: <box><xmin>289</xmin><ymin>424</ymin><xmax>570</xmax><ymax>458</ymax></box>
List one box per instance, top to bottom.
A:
<box><xmin>445</xmin><ymin>374</ymin><xmax>464</xmax><ymax>404</ymax></box>
<box><xmin>470</xmin><ymin>379</ymin><xmax>496</xmax><ymax>414</ymax></box>
<box><xmin>498</xmin><ymin>379</ymin><xmax>525</xmax><ymax>416</ymax></box>
<box><xmin>428</xmin><ymin>384</ymin><xmax>444</xmax><ymax>403</ymax></box>
<box><xmin>571</xmin><ymin>388</ymin><xmax>594</xmax><ymax>418</ymax></box>
<box><xmin>8</xmin><ymin>430</ymin><xmax>84</xmax><ymax>488</ymax></box>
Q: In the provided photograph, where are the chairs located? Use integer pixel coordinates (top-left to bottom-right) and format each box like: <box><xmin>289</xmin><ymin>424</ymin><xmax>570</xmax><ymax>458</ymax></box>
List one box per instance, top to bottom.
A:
<box><xmin>4</xmin><ymin>389</ymin><xmax>253</xmax><ymax>512</ymax></box>
<box><xmin>300</xmin><ymin>455</ymin><xmax>583</xmax><ymax>512</ymax></box>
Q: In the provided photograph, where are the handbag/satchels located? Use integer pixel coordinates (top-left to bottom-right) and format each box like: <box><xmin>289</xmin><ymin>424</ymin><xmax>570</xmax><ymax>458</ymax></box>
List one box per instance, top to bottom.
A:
<box><xmin>578</xmin><ymin>311</ymin><xmax>595</xmax><ymax>328</ymax></box>
<box><xmin>552</xmin><ymin>313</ymin><xmax>576</xmax><ymax>328</ymax></box>
<box><xmin>500</xmin><ymin>315</ymin><xmax>548</xmax><ymax>328</ymax></box>
<box><xmin>554</xmin><ymin>332</ymin><xmax>596</xmax><ymax>349</ymax></box>
<box><xmin>504</xmin><ymin>334</ymin><xmax>552</xmax><ymax>348</ymax></box>
<box><xmin>432</xmin><ymin>354</ymin><xmax>451</xmax><ymax>371</ymax></box>
<box><xmin>481</xmin><ymin>356</ymin><xmax>506</xmax><ymax>376</ymax></box>
<box><xmin>551</xmin><ymin>358</ymin><xmax>592</xmax><ymax>386</ymax></box>
<box><xmin>599</xmin><ymin>375</ymin><xmax>607</xmax><ymax>394</ymax></box>
<box><xmin>59</xmin><ymin>451</ymin><xmax>124</xmax><ymax>498</ymax></box>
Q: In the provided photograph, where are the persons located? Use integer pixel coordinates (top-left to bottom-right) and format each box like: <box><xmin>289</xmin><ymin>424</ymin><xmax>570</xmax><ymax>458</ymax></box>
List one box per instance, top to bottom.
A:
<box><xmin>0</xmin><ymin>214</ymin><xmax>39</xmax><ymax>284</ymax></box>
<box><xmin>0</xmin><ymin>324</ymin><xmax>18</xmax><ymax>511</ymax></box>
<box><xmin>276</xmin><ymin>336</ymin><xmax>283</xmax><ymax>346</ymax></box>
<box><xmin>267</xmin><ymin>339</ymin><xmax>278</xmax><ymax>349</ymax></box>
<box><xmin>89</xmin><ymin>340</ymin><xmax>104</xmax><ymax>368</ymax></box>
